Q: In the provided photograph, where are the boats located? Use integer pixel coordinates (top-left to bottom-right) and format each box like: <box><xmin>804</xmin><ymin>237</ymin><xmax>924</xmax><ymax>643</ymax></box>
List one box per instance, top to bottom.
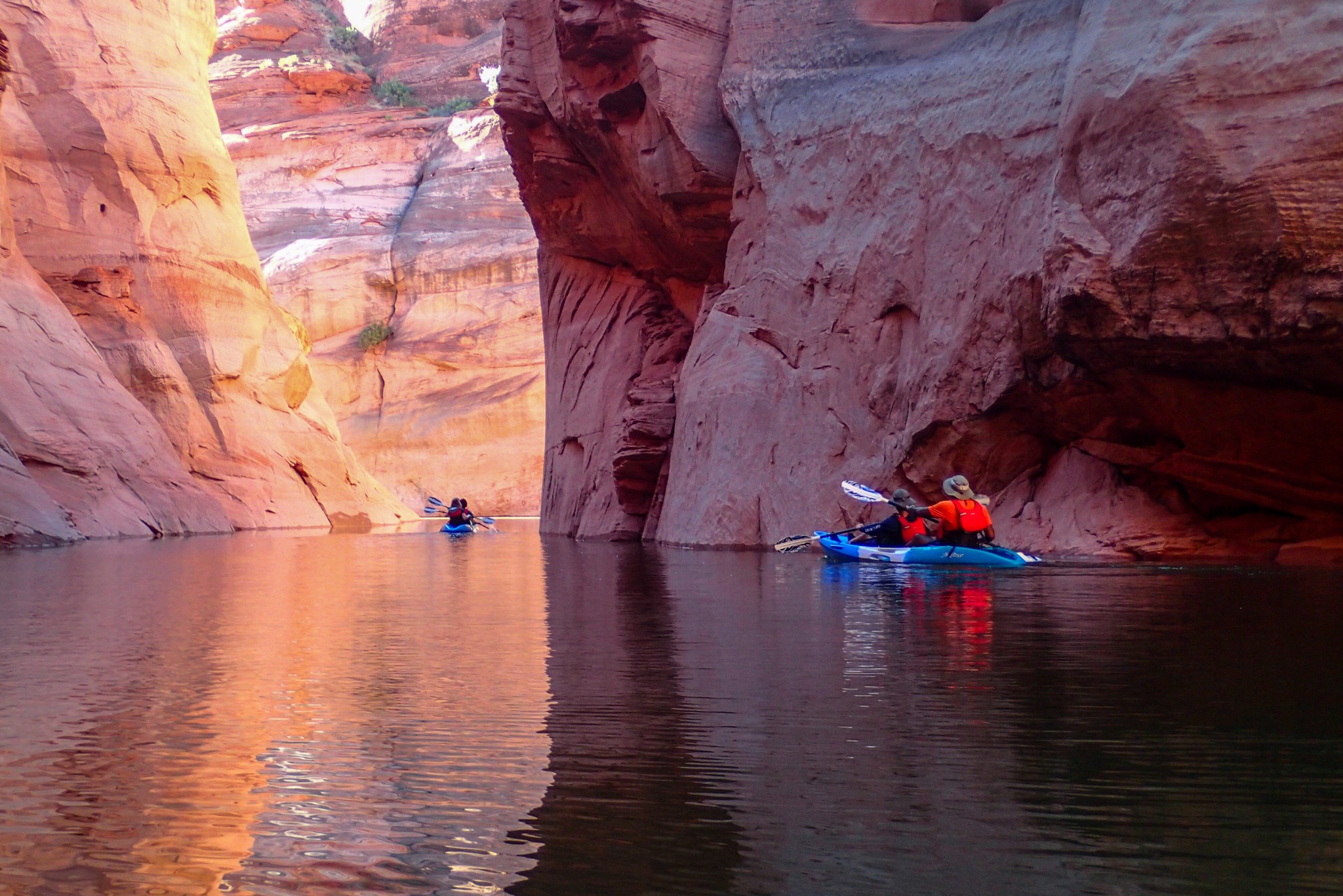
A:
<box><xmin>439</xmin><ymin>519</ymin><xmax>479</xmax><ymax>533</ymax></box>
<box><xmin>813</xmin><ymin>528</ymin><xmax>1042</xmax><ymax>571</ymax></box>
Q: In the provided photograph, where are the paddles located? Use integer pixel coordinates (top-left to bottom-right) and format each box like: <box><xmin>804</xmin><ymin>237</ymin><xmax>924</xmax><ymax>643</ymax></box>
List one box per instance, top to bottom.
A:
<box><xmin>840</xmin><ymin>480</ymin><xmax>1042</xmax><ymax>562</ymax></box>
<box><xmin>774</xmin><ymin>493</ymin><xmax>990</xmax><ymax>554</ymax></box>
<box><xmin>427</xmin><ymin>496</ymin><xmax>500</xmax><ymax>532</ymax></box>
<box><xmin>423</xmin><ymin>507</ymin><xmax>495</xmax><ymax>525</ymax></box>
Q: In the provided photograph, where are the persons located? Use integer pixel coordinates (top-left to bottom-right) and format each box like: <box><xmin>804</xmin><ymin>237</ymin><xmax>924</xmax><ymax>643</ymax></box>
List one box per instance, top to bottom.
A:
<box><xmin>905</xmin><ymin>475</ymin><xmax>995</xmax><ymax>549</ymax></box>
<box><xmin>831</xmin><ymin>489</ymin><xmax>930</xmax><ymax>547</ymax></box>
<box><xmin>445</xmin><ymin>497</ymin><xmax>471</xmax><ymax>526</ymax></box>
<box><xmin>459</xmin><ymin>498</ymin><xmax>481</xmax><ymax>524</ymax></box>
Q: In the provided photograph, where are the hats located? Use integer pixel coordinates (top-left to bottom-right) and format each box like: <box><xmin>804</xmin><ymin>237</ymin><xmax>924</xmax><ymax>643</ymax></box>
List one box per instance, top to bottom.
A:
<box><xmin>943</xmin><ymin>475</ymin><xmax>974</xmax><ymax>500</ymax></box>
<box><xmin>887</xmin><ymin>489</ymin><xmax>915</xmax><ymax>507</ymax></box>
<box><xmin>450</xmin><ymin>497</ymin><xmax>461</xmax><ymax>507</ymax></box>
<box><xmin>460</xmin><ymin>498</ymin><xmax>467</xmax><ymax>508</ymax></box>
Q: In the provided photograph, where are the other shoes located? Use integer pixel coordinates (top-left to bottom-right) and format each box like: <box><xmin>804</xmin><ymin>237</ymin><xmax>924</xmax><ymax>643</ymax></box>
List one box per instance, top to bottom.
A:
<box><xmin>831</xmin><ymin>531</ymin><xmax>841</xmax><ymax>542</ymax></box>
<box><xmin>848</xmin><ymin>533</ymin><xmax>856</xmax><ymax>543</ymax></box>
